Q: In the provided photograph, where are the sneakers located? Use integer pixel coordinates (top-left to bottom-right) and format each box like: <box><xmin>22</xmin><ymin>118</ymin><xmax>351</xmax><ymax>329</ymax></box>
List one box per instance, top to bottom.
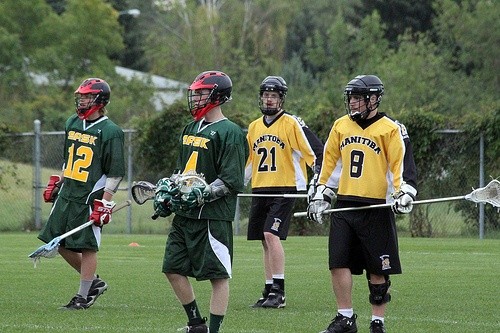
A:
<box><xmin>86</xmin><ymin>275</ymin><xmax>108</xmax><ymax>307</ymax></box>
<box><xmin>260</xmin><ymin>284</ymin><xmax>286</xmax><ymax>308</ymax></box>
<box><xmin>56</xmin><ymin>294</ymin><xmax>87</xmax><ymax>310</ymax></box>
<box><xmin>249</xmin><ymin>297</ymin><xmax>267</xmax><ymax>308</ymax></box>
<box><xmin>319</xmin><ymin>312</ymin><xmax>357</xmax><ymax>333</ymax></box>
<box><xmin>176</xmin><ymin>317</ymin><xmax>209</xmax><ymax>333</ymax></box>
<box><xmin>368</xmin><ymin>319</ymin><xmax>384</xmax><ymax>333</ymax></box>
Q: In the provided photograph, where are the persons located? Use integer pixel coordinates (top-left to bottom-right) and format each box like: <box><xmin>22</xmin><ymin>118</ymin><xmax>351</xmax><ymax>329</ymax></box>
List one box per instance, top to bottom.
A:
<box><xmin>152</xmin><ymin>70</ymin><xmax>244</xmax><ymax>333</ymax></box>
<box><xmin>238</xmin><ymin>74</ymin><xmax>324</xmax><ymax>309</ymax></box>
<box><xmin>307</xmin><ymin>74</ymin><xmax>417</xmax><ymax>333</ymax></box>
<box><xmin>42</xmin><ymin>78</ymin><xmax>125</xmax><ymax>310</ymax></box>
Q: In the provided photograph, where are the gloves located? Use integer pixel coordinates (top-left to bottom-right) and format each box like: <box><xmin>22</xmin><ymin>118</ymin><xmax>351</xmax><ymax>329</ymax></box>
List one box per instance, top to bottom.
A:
<box><xmin>43</xmin><ymin>175</ymin><xmax>62</xmax><ymax>202</ymax></box>
<box><xmin>154</xmin><ymin>177</ymin><xmax>179</xmax><ymax>217</ymax></box>
<box><xmin>181</xmin><ymin>178</ymin><xmax>231</xmax><ymax>211</ymax></box>
<box><xmin>307</xmin><ymin>181</ymin><xmax>318</xmax><ymax>204</ymax></box>
<box><xmin>391</xmin><ymin>183</ymin><xmax>418</xmax><ymax>214</ymax></box>
<box><xmin>307</xmin><ymin>186</ymin><xmax>336</xmax><ymax>224</ymax></box>
<box><xmin>90</xmin><ymin>199</ymin><xmax>115</xmax><ymax>226</ymax></box>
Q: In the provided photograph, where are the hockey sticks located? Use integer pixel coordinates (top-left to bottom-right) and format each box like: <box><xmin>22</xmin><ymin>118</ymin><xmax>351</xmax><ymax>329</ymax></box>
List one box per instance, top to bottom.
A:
<box><xmin>293</xmin><ymin>179</ymin><xmax>500</xmax><ymax>217</ymax></box>
<box><xmin>131</xmin><ymin>181</ymin><xmax>308</xmax><ymax>205</ymax></box>
<box><xmin>29</xmin><ymin>200</ymin><xmax>132</xmax><ymax>258</ymax></box>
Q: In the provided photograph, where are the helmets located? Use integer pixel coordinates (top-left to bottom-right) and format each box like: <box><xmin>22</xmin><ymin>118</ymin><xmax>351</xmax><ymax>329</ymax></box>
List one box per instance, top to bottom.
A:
<box><xmin>187</xmin><ymin>71</ymin><xmax>233</xmax><ymax>120</ymax></box>
<box><xmin>343</xmin><ymin>75</ymin><xmax>384</xmax><ymax>121</ymax></box>
<box><xmin>258</xmin><ymin>76</ymin><xmax>288</xmax><ymax>116</ymax></box>
<box><xmin>74</xmin><ymin>77</ymin><xmax>111</xmax><ymax>119</ymax></box>
